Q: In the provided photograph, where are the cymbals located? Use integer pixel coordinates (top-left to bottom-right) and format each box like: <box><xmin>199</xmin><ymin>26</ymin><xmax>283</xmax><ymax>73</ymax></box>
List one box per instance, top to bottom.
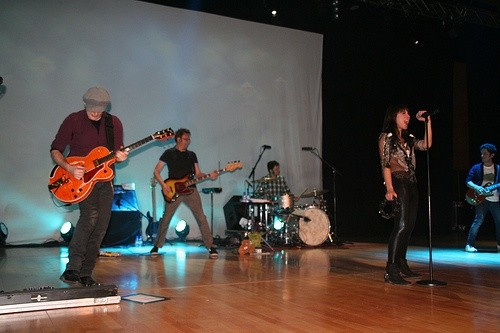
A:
<box><xmin>251</xmin><ymin>177</ymin><xmax>282</xmax><ymax>183</ymax></box>
<box><xmin>302</xmin><ymin>190</ymin><xmax>328</xmax><ymax>198</ymax></box>
<box><xmin>250</xmin><ymin>191</ymin><xmax>273</xmax><ymax>195</ymax></box>
<box><xmin>377</xmin><ymin>196</ymin><xmax>401</xmax><ymax>219</ymax></box>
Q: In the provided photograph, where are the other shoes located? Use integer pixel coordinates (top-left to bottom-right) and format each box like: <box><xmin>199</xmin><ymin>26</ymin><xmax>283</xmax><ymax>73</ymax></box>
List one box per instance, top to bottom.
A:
<box><xmin>465</xmin><ymin>244</ymin><xmax>477</xmax><ymax>252</ymax></box>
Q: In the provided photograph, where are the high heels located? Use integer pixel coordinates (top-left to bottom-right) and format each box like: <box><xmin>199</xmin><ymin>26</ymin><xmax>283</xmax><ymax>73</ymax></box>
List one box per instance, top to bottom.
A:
<box><xmin>399</xmin><ymin>259</ymin><xmax>421</xmax><ymax>277</ymax></box>
<box><xmin>385</xmin><ymin>262</ymin><xmax>411</xmax><ymax>285</ymax></box>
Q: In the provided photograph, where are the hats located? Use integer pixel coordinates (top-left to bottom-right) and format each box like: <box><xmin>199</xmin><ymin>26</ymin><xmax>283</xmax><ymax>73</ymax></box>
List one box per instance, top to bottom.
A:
<box><xmin>82</xmin><ymin>87</ymin><xmax>109</xmax><ymax>112</ymax></box>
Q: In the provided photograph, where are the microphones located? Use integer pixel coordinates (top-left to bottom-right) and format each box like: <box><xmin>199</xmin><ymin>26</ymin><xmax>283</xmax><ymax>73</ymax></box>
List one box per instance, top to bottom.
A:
<box><xmin>415</xmin><ymin>109</ymin><xmax>439</xmax><ymax>118</ymax></box>
<box><xmin>261</xmin><ymin>144</ymin><xmax>271</xmax><ymax>148</ymax></box>
<box><xmin>302</xmin><ymin>147</ymin><xmax>315</xmax><ymax>150</ymax></box>
<box><xmin>116</xmin><ymin>192</ymin><xmax>121</xmax><ymax>205</ymax></box>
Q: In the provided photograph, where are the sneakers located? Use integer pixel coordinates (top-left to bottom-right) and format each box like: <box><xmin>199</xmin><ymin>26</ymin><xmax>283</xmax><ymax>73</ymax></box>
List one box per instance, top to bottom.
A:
<box><xmin>150</xmin><ymin>246</ymin><xmax>158</xmax><ymax>254</ymax></box>
<box><xmin>208</xmin><ymin>247</ymin><xmax>218</xmax><ymax>256</ymax></box>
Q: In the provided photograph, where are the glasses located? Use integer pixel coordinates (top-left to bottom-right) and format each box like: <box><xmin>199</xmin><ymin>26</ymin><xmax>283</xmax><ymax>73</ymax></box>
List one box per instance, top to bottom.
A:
<box><xmin>181</xmin><ymin>138</ymin><xmax>191</xmax><ymax>141</ymax></box>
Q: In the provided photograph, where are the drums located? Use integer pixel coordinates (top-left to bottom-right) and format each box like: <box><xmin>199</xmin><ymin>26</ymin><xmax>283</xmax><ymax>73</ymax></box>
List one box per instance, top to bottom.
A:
<box><xmin>277</xmin><ymin>194</ymin><xmax>295</xmax><ymax>214</ymax></box>
<box><xmin>247</xmin><ymin>199</ymin><xmax>272</xmax><ymax>227</ymax></box>
<box><xmin>286</xmin><ymin>206</ymin><xmax>331</xmax><ymax>247</ymax></box>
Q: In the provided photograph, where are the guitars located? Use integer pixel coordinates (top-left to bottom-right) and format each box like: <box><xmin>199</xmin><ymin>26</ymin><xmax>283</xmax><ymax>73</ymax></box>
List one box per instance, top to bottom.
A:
<box><xmin>48</xmin><ymin>127</ymin><xmax>176</xmax><ymax>204</ymax></box>
<box><xmin>161</xmin><ymin>160</ymin><xmax>243</xmax><ymax>203</ymax></box>
<box><xmin>145</xmin><ymin>177</ymin><xmax>163</xmax><ymax>243</ymax></box>
<box><xmin>465</xmin><ymin>181</ymin><xmax>500</xmax><ymax>206</ymax></box>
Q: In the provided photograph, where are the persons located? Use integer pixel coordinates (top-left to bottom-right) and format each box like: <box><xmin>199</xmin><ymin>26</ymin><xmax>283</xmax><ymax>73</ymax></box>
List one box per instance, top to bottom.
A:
<box><xmin>49</xmin><ymin>86</ymin><xmax>130</xmax><ymax>287</ymax></box>
<box><xmin>379</xmin><ymin>103</ymin><xmax>433</xmax><ymax>285</ymax></box>
<box><xmin>150</xmin><ymin>129</ymin><xmax>218</xmax><ymax>255</ymax></box>
<box><xmin>465</xmin><ymin>143</ymin><xmax>500</xmax><ymax>252</ymax></box>
<box><xmin>254</xmin><ymin>161</ymin><xmax>300</xmax><ymax>225</ymax></box>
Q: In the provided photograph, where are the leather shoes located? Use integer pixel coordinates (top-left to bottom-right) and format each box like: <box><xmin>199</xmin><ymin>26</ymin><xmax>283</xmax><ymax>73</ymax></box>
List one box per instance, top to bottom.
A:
<box><xmin>60</xmin><ymin>272</ymin><xmax>78</xmax><ymax>284</ymax></box>
<box><xmin>78</xmin><ymin>276</ymin><xmax>100</xmax><ymax>287</ymax></box>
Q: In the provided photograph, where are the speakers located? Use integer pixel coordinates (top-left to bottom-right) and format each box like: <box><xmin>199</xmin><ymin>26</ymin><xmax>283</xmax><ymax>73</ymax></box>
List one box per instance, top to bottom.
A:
<box><xmin>110</xmin><ymin>189</ymin><xmax>141</xmax><ymax>222</ymax></box>
<box><xmin>224</xmin><ymin>195</ymin><xmax>250</xmax><ymax>230</ymax></box>
